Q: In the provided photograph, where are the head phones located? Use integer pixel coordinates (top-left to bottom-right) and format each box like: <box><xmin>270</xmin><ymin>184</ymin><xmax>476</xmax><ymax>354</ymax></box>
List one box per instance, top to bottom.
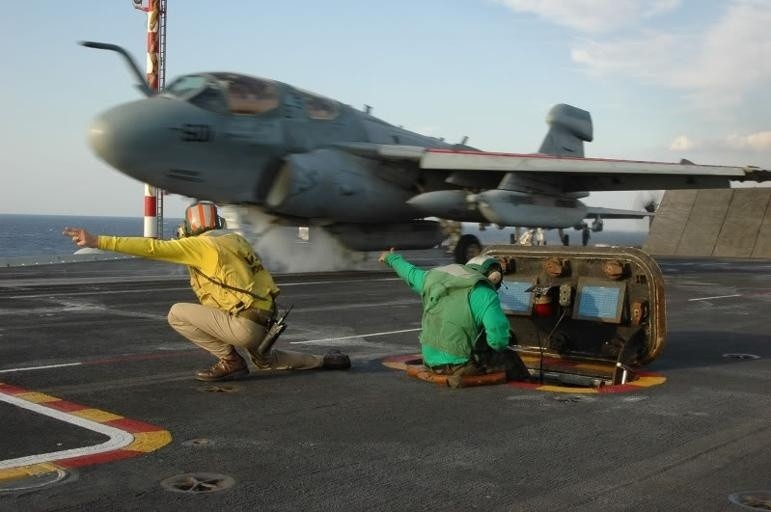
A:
<box><xmin>488</xmin><ymin>267</ymin><xmax>502</xmax><ymax>286</ymax></box>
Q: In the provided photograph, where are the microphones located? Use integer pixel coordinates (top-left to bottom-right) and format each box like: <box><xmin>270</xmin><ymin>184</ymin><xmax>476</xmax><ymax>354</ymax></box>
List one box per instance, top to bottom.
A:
<box><xmin>500</xmin><ymin>279</ymin><xmax>509</xmax><ymax>292</ymax></box>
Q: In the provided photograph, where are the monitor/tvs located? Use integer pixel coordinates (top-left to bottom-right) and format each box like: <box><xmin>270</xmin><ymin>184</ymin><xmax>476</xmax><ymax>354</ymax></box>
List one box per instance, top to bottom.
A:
<box><xmin>496</xmin><ymin>275</ymin><xmax>538</xmax><ymax>317</ymax></box>
<box><xmin>572</xmin><ymin>275</ymin><xmax>627</xmax><ymax>324</ymax></box>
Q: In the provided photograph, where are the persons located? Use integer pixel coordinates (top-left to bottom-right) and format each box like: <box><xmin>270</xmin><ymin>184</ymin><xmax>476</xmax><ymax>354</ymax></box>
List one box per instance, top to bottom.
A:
<box><xmin>61</xmin><ymin>201</ymin><xmax>351</xmax><ymax>381</ymax></box>
<box><xmin>379</xmin><ymin>245</ymin><xmax>531</xmax><ymax>382</ymax></box>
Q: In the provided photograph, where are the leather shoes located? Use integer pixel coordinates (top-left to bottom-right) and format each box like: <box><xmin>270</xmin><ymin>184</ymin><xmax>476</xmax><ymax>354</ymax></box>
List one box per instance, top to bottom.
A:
<box><xmin>323</xmin><ymin>349</ymin><xmax>351</xmax><ymax>368</ymax></box>
<box><xmin>194</xmin><ymin>350</ymin><xmax>248</xmax><ymax>381</ymax></box>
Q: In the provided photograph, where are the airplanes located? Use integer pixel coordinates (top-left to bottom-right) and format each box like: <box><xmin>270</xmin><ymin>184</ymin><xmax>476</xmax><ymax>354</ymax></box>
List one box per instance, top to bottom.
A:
<box><xmin>85</xmin><ymin>67</ymin><xmax>771</xmax><ymax>253</ymax></box>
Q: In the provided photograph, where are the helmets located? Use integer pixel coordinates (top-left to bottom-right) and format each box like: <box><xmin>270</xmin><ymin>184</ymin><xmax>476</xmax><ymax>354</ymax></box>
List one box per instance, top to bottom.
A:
<box><xmin>466</xmin><ymin>255</ymin><xmax>503</xmax><ymax>290</ymax></box>
